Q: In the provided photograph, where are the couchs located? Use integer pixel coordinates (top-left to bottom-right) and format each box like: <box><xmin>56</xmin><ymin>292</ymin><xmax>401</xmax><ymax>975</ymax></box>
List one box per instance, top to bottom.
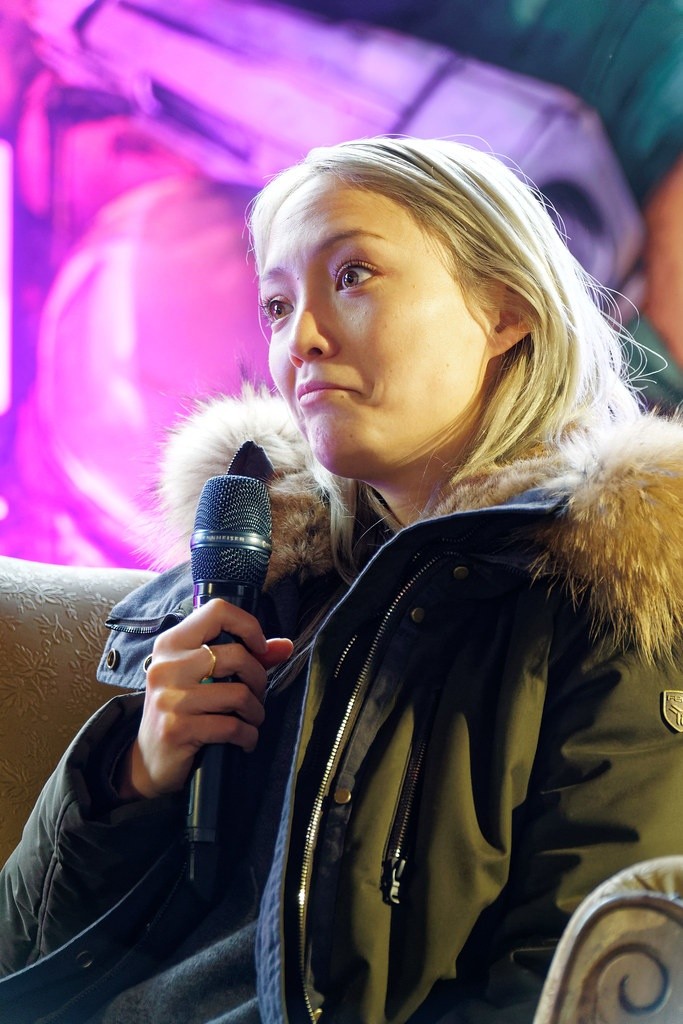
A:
<box><xmin>1</xmin><ymin>554</ymin><xmax>683</xmax><ymax>1024</ymax></box>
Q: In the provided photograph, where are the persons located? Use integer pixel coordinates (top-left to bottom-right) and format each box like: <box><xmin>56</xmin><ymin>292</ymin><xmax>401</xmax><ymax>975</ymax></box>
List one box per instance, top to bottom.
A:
<box><xmin>1</xmin><ymin>138</ymin><xmax>683</xmax><ymax>1023</ymax></box>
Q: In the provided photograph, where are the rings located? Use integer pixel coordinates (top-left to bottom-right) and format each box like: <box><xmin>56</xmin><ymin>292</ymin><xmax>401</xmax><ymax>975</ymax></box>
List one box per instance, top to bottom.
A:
<box><xmin>201</xmin><ymin>643</ymin><xmax>218</xmax><ymax>680</ymax></box>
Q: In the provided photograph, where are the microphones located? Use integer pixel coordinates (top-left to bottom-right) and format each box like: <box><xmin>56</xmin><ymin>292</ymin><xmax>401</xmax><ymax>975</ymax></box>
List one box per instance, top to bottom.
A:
<box><xmin>183</xmin><ymin>474</ymin><xmax>272</xmax><ymax>894</ymax></box>
